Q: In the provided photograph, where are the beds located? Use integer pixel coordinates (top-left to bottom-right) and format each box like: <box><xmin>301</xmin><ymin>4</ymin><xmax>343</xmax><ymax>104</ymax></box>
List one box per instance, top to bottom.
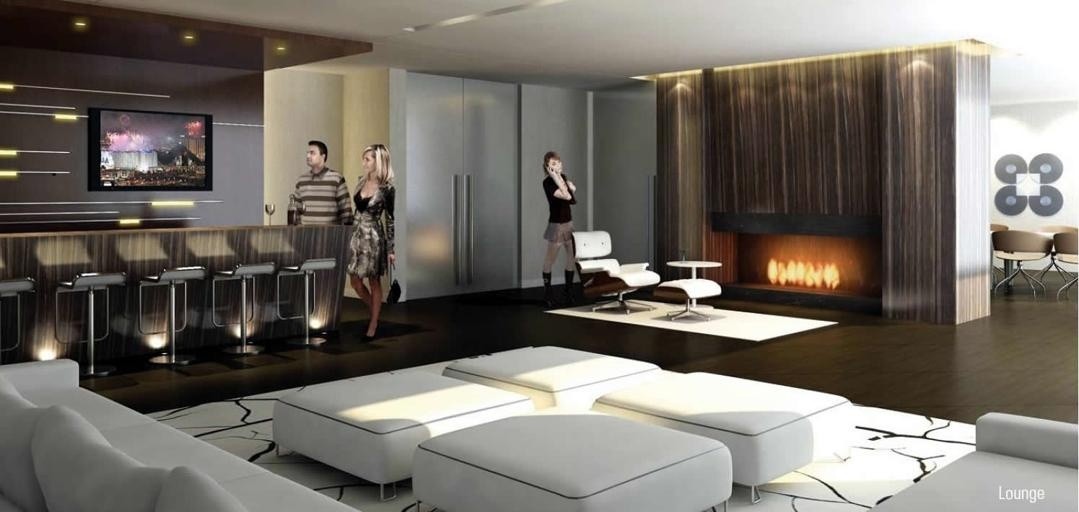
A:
<box><xmin>668</xmin><ymin>260</ymin><xmax>723</xmax><ymax>312</ymax></box>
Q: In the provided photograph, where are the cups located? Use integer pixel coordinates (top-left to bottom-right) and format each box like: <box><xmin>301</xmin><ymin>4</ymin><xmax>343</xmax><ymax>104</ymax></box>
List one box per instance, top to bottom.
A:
<box><xmin>296</xmin><ymin>201</ymin><xmax>306</xmax><ymax>224</ymax></box>
<box><xmin>265</xmin><ymin>203</ymin><xmax>275</xmax><ymax>226</ymax></box>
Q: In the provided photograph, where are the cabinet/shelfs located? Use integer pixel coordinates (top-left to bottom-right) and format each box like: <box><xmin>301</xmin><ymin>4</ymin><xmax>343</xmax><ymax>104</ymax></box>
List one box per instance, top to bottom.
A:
<box><xmin>405</xmin><ymin>71</ymin><xmax>521</xmax><ymax>303</ymax></box>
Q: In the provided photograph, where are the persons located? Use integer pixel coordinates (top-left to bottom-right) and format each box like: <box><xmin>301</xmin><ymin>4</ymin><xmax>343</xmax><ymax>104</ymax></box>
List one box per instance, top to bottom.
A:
<box><xmin>292</xmin><ymin>141</ymin><xmax>354</xmax><ymax>226</ymax></box>
<box><xmin>346</xmin><ymin>144</ymin><xmax>396</xmax><ymax>342</ymax></box>
<box><xmin>543</xmin><ymin>151</ymin><xmax>577</xmax><ymax>309</ymax></box>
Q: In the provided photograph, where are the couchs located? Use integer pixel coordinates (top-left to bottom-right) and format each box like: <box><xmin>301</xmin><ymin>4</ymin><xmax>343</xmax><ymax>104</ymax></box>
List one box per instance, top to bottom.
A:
<box><xmin>443</xmin><ymin>346</ymin><xmax>661</xmax><ymax>411</ymax></box>
<box><xmin>0</xmin><ymin>359</ymin><xmax>364</xmax><ymax>512</ymax></box>
<box><xmin>274</xmin><ymin>368</ymin><xmax>535</xmax><ymax>501</ymax></box>
<box><xmin>590</xmin><ymin>370</ymin><xmax>855</xmax><ymax>504</ymax></box>
<box><xmin>411</xmin><ymin>407</ymin><xmax>733</xmax><ymax>512</ymax></box>
<box><xmin>869</xmin><ymin>413</ymin><xmax>1076</xmax><ymax>509</ymax></box>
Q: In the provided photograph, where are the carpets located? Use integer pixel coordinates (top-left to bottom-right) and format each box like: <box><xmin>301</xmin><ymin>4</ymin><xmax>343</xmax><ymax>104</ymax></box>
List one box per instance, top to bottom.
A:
<box><xmin>538</xmin><ymin>298</ymin><xmax>840</xmax><ymax>342</ymax></box>
<box><xmin>144</xmin><ymin>344</ymin><xmax>977</xmax><ymax>512</ymax></box>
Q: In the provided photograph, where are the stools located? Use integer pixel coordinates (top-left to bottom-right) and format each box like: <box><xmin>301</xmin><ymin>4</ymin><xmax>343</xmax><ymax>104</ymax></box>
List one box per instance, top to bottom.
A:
<box><xmin>275</xmin><ymin>257</ymin><xmax>338</xmax><ymax>346</ymax></box>
<box><xmin>213</xmin><ymin>262</ymin><xmax>276</xmax><ymax>356</ymax></box>
<box><xmin>138</xmin><ymin>266</ymin><xmax>205</xmax><ymax>365</ymax></box>
<box><xmin>53</xmin><ymin>272</ymin><xmax>127</xmax><ymax>377</ymax></box>
<box><xmin>0</xmin><ymin>276</ymin><xmax>35</xmax><ymax>352</ymax></box>
<box><xmin>659</xmin><ymin>279</ymin><xmax>721</xmax><ymax>322</ymax></box>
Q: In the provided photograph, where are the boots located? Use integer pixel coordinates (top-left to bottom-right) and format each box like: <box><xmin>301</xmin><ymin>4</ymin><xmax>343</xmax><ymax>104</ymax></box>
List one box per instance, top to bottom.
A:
<box><xmin>562</xmin><ymin>271</ymin><xmax>576</xmax><ymax>305</ymax></box>
<box><xmin>543</xmin><ymin>272</ymin><xmax>561</xmax><ymax>308</ymax></box>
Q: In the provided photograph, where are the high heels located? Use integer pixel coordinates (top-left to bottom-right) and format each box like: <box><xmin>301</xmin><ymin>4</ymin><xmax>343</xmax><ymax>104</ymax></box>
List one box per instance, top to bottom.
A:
<box><xmin>363</xmin><ymin>319</ymin><xmax>380</xmax><ymax>343</ymax></box>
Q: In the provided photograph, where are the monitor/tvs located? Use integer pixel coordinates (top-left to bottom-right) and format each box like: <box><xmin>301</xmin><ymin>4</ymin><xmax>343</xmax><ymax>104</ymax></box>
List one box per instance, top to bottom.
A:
<box><xmin>86</xmin><ymin>106</ymin><xmax>213</xmax><ymax>192</ymax></box>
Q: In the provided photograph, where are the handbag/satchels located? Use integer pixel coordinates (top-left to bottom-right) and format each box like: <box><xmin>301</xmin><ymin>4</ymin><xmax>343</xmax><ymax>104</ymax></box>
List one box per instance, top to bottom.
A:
<box><xmin>387</xmin><ymin>257</ymin><xmax>402</xmax><ymax>306</ymax></box>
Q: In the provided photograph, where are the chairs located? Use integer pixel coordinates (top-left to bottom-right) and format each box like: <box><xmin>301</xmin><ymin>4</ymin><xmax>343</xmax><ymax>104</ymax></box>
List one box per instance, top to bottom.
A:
<box><xmin>992</xmin><ymin>223</ymin><xmax>1014</xmax><ymax>289</ymax></box>
<box><xmin>1029</xmin><ymin>227</ymin><xmax>1078</xmax><ymax>289</ymax></box>
<box><xmin>570</xmin><ymin>230</ymin><xmax>660</xmax><ymax>314</ymax></box>
<box><xmin>991</xmin><ymin>230</ymin><xmax>1054</xmax><ymax>298</ymax></box>
<box><xmin>1052</xmin><ymin>231</ymin><xmax>1079</xmax><ymax>302</ymax></box>
